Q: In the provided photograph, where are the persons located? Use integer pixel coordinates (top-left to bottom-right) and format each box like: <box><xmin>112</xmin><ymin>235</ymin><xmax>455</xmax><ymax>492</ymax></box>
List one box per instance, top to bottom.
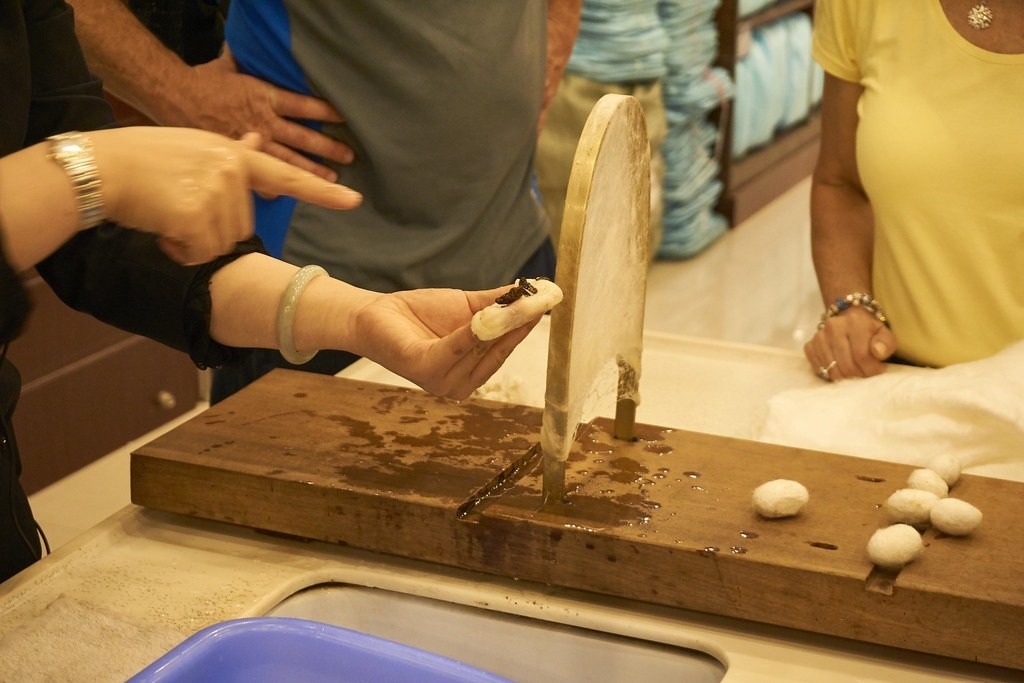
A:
<box><xmin>0</xmin><ymin>0</ymin><xmax>546</xmax><ymax>584</ymax></box>
<box><xmin>61</xmin><ymin>0</ymin><xmax>582</xmax><ymax>406</ymax></box>
<box><xmin>758</xmin><ymin>0</ymin><xmax>1023</xmax><ymax>386</ymax></box>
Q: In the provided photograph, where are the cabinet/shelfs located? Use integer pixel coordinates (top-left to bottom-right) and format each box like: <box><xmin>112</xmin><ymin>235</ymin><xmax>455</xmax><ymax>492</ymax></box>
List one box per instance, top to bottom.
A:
<box><xmin>704</xmin><ymin>1</ymin><xmax>827</xmax><ymax>230</ymax></box>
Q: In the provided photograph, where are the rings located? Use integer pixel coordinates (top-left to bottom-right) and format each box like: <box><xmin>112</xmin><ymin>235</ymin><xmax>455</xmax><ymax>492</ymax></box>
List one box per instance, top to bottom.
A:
<box><xmin>819</xmin><ymin>360</ymin><xmax>836</xmax><ymax>379</ymax></box>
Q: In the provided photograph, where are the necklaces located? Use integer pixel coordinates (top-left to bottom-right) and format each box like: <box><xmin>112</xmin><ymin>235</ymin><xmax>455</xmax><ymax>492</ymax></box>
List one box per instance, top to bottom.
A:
<box><xmin>966</xmin><ymin>0</ymin><xmax>993</xmax><ymax>29</ymax></box>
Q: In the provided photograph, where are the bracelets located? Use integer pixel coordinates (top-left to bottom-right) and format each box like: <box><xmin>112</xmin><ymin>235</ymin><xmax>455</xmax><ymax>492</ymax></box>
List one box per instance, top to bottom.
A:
<box><xmin>275</xmin><ymin>264</ymin><xmax>331</xmax><ymax>365</ymax></box>
<box><xmin>816</xmin><ymin>290</ymin><xmax>886</xmax><ymax>329</ymax></box>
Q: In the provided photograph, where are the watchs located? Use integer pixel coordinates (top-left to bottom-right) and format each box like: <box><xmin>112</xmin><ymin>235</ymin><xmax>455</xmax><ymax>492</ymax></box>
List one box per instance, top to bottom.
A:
<box><xmin>44</xmin><ymin>129</ymin><xmax>107</xmax><ymax>234</ymax></box>
